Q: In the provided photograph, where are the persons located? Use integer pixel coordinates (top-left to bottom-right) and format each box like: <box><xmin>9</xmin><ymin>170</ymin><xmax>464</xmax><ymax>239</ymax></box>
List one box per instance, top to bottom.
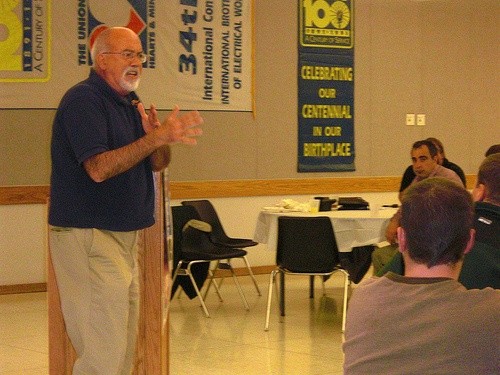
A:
<box><xmin>48</xmin><ymin>26</ymin><xmax>203</xmax><ymax>375</ymax></box>
<box><xmin>372</xmin><ymin>137</ymin><xmax>500</xmax><ymax>290</ymax></box>
<box><xmin>342</xmin><ymin>176</ymin><xmax>500</xmax><ymax>375</ymax></box>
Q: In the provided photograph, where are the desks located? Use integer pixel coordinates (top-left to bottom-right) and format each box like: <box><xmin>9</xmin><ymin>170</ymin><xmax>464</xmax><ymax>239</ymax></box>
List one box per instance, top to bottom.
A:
<box><xmin>254</xmin><ymin>205</ymin><xmax>399</xmax><ymax>316</ymax></box>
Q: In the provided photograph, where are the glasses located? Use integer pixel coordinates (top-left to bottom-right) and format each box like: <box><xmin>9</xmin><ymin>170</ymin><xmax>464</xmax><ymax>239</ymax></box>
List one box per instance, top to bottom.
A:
<box><xmin>101</xmin><ymin>49</ymin><xmax>148</xmax><ymax>63</ymax></box>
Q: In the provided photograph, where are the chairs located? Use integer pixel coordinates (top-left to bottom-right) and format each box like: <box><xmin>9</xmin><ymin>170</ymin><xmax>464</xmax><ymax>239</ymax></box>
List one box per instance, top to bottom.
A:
<box><xmin>167</xmin><ymin>200</ymin><xmax>259</xmax><ymax>317</ymax></box>
<box><xmin>263</xmin><ymin>215</ymin><xmax>349</xmax><ymax>332</ymax></box>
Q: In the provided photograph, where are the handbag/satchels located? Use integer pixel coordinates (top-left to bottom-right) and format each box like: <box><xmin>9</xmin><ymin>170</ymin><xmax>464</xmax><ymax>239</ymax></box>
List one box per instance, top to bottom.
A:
<box><xmin>338</xmin><ymin>197</ymin><xmax>369</xmax><ymax>209</ymax></box>
